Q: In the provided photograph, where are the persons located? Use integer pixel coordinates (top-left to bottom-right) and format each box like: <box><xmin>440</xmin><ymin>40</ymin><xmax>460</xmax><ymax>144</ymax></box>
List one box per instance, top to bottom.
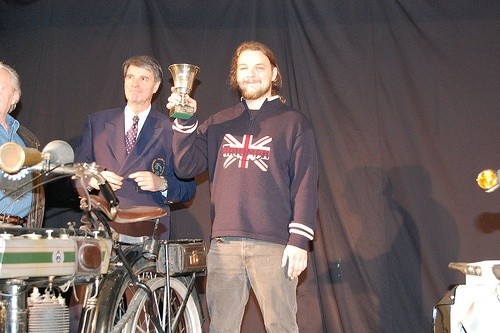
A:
<box><xmin>167</xmin><ymin>42</ymin><xmax>320</xmax><ymax>333</ymax></box>
<box><xmin>77</xmin><ymin>55</ymin><xmax>195</xmax><ymax>242</ymax></box>
<box><xmin>0</xmin><ymin>62</ymin><xmax>46</xmax><ymax>229</ymax></box>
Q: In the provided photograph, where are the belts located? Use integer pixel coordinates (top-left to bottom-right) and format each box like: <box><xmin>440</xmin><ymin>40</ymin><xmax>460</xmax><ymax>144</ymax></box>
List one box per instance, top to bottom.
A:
<box><xmin>0</xmin><ymin>214</ymin><xmax>26</xmax><ymax>225</ymax></box>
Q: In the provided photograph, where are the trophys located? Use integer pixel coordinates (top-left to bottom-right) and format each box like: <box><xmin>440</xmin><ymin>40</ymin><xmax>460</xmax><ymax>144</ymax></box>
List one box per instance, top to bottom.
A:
<box><xmin>167</xmin><ymin>63</ymin><xmax>200</xmax><ymax>120</ymax></box>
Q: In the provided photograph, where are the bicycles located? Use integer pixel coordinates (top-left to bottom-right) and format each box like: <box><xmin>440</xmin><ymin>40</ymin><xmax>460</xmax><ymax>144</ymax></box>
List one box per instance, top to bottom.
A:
<box><xmin>0</xmin><ymin>140</ymin><xmax>208</xmax><ymax>333</ymax></box>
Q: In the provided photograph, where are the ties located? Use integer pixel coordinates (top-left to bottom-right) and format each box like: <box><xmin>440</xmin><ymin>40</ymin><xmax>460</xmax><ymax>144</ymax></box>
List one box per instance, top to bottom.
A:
<box><xmin>125</xmin><ymin>115</ymin><xmax>140</xmax><ymax>156</ymax></box>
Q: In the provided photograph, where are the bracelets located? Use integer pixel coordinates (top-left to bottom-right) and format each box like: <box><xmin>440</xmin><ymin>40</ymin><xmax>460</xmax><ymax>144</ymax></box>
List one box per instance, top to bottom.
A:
<box><xmin>160</xmin><ymin>176</ymin><xmax>168</xmax><ymax>192</ymax></box>
